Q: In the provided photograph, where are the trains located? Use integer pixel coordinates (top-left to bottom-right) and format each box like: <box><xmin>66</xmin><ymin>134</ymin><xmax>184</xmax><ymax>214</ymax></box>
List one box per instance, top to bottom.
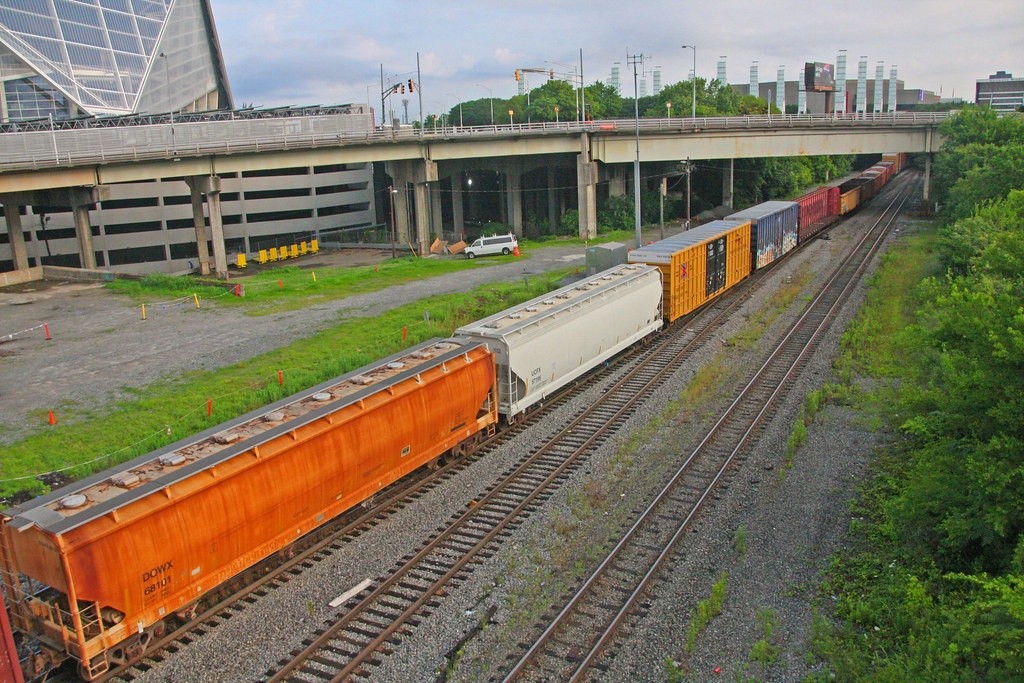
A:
<box><xmin>0</xmin><ymin>151</ymin><xmax>915</xmax><ymax>683</ymax></box>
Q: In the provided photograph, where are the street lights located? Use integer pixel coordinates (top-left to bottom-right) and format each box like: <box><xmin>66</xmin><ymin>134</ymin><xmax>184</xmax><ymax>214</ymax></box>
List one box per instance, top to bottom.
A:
<box><xmin>682</xmin><ymin>45</ymin><xmax>697</xmax><ymax>118</ymax></box>
<box><xmin>544</xmin><ymin>60</ymin><xmax>579</xmax><ymax>121</ymax></box>
<box><xmin>387</xmin><ymin>77</ymin><xmax>392</xmax><ymax>125</ymax></box>
<box><xmin>666</xmin><ymin>103</ymin><xmax>672</xmax><ymax>126</ymax></box>
<box><xmin>555</xmin><ymin>106</ymin><xmax>559</xmax><ymax>122</ymax></box>
<box><xmin>508</xmin><ymin>110</ymin><xmax>514</xmax><ymax>129</ymax></box>
<box><xmin>389</xmin><ymin>185</ymin><xmax>398</xmax><ymax>257</ymax></box>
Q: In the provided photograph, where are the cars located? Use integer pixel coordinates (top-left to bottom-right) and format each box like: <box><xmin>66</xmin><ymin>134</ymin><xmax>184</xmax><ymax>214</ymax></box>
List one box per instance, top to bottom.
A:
<box><xmin>463</xmin><ymin>231</ymin><xmax>519</xmax><ymax>259</ymax></box>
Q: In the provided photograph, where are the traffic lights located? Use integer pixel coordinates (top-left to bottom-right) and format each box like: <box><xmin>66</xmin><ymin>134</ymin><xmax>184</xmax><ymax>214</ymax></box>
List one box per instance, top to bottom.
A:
<box><xmin>549</xmin><ymin>68</ymin><xmax>554</xmax><ymax>79</ymax></box>
<box><xmin>515</xmin><ymin>71</ymin><xmax>519</xmax><ymax>81</ymax></box>
<box><xmin>408</xmin><ymin>79</ymin><xmax>413</xmax><ymax>92</ymax></box>
<box><xmin>401</xmin><ymin>84</ymin><xmax>404</xmax><ymax>94</ymax></box>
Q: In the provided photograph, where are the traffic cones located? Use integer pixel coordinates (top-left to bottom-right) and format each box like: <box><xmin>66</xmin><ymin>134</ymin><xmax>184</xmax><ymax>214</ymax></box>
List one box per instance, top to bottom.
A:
<box><xmin>513</xmin><ymin>246</ymin><xmax>520</xmax><ymax>256</ymax></box>
